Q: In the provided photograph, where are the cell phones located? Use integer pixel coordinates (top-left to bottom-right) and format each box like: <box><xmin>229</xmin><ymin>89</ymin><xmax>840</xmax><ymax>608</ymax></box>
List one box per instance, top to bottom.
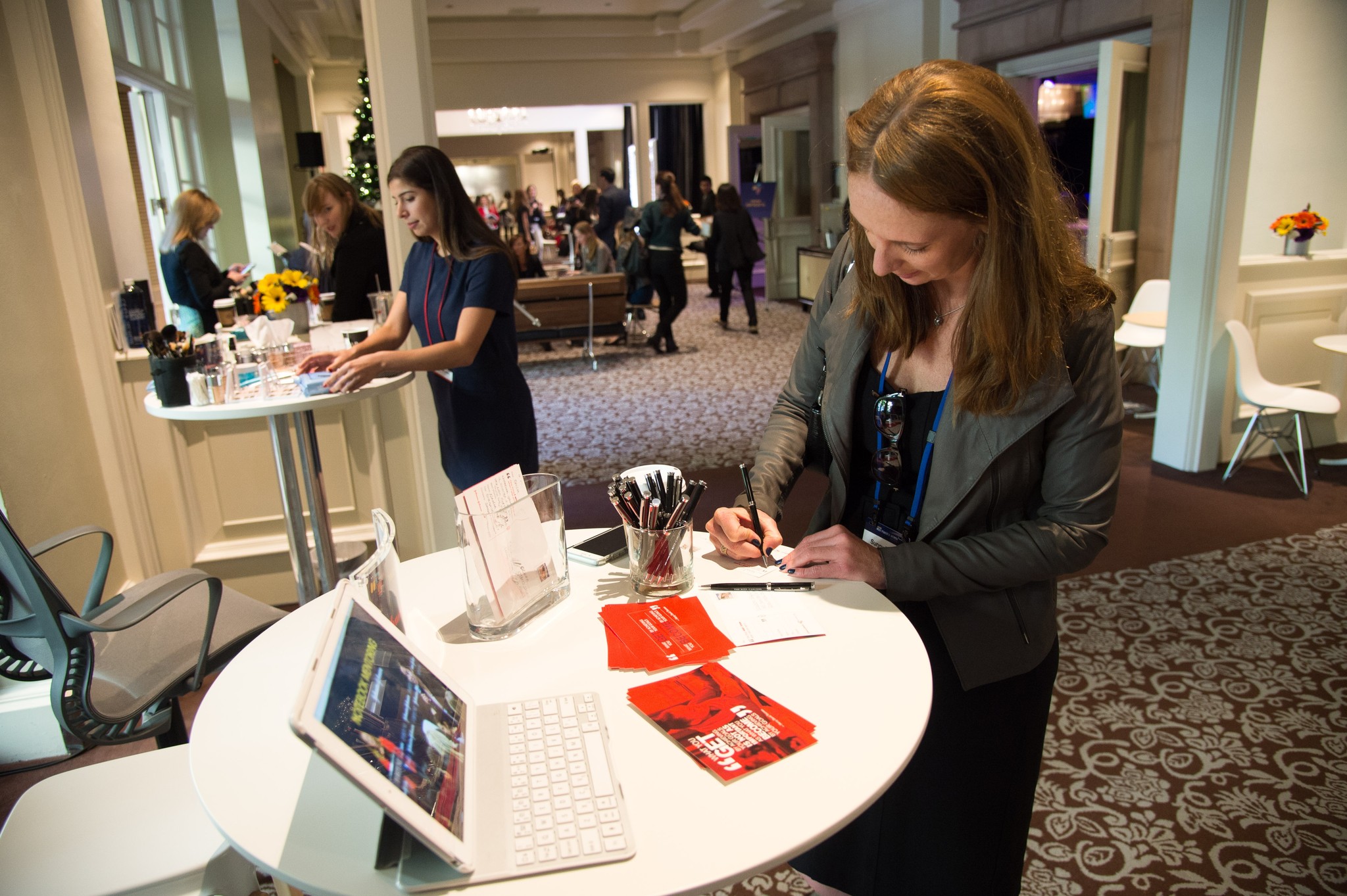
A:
<box><xmin>568</xmin><ymin>521</ymin><xmax>628</xmax><ymax>566</ymax></box>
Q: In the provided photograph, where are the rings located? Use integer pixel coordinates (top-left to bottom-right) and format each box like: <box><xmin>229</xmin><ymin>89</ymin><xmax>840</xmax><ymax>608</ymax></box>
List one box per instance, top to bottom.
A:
<box><xmin>720</xmin><ymin>545</ymin><xmax>728</xmax><ymax>556</ymax></box>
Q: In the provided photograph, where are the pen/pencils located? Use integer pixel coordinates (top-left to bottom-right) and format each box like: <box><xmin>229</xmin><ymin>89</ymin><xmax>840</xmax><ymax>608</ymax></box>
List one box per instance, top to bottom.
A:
<box><xmin>737</xmin><ymin>464</ymin><xmax>770</xmax><ymax>568</ymax></box>
<box><xmin>607</xmin><ymin>471</ymin><xmax>708</xmax><ymax>585</ymax></box>
<box><xmin>701</xmin><ymin>581</ymin><xmax>815</xmax><ymax>593</ymax></box>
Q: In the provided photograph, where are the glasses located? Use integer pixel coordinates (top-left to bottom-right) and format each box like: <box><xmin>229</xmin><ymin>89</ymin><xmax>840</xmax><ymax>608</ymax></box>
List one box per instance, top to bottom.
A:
<box><xmin>872</xmin><ymin>387</ymin><xmax>908</xmax><ymax>497</ymax></box>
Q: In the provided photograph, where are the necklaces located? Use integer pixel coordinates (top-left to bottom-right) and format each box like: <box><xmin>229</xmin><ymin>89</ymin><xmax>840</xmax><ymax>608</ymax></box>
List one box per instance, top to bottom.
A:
<box><xmin>931</xmin><ymin>304</ymin><xmax>965</xmax><ymax>326</ymax></box>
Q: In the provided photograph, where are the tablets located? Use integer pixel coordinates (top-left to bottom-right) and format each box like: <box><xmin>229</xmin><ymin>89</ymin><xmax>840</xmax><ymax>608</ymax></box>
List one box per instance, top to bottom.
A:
<box><xmin>293</xmin><ymin>578</ymin><xmax>473</xmax><ymax>872</ymax></box>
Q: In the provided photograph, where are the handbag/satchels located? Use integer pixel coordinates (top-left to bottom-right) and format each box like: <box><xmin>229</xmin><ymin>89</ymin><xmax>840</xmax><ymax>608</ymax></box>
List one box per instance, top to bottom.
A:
<box><xmin>803</xmin><ymin>385</ymin><xmax>833</xmax><ymax>479</ymax></box>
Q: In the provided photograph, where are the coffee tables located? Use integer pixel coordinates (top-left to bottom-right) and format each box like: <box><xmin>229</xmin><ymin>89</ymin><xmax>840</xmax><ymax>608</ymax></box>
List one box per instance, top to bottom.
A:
<box><xmin>1310</xmin><ymin>333</ymin><xmax>1347</xmax><ymax>467</ymax></box>
<box><xmin>141</xmin><ymin>367</ymin><xmax>416</xmax><ymax>609</ymax></box>
<box><xmin>181</xmin><ymin>526</ymin><xmax>933</xmax><ymax>896</ymax></box>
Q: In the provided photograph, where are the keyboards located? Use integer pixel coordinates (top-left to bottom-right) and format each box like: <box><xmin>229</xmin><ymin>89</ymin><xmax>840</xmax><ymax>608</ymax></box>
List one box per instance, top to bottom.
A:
<box><xmin>504</xmin><ymin>694</ymin><xmax>631</xmax><ymax>867</ymax></box>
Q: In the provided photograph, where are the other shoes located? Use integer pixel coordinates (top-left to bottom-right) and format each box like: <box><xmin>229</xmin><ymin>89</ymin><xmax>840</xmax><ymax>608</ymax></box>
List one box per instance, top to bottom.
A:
<box><xmin>713</xmin><ymin>317</ymin><xmax>729</xmax><ymax>330</ymax></box>
<box><xmin>748</xmin><ymin>325</ymin><xmax>758</xmax><ymax>335</ymax></box>
<box><xmin>646</xmin><ymin>336</ymin><xmax>679</xmax><ymax>355</ymax></box>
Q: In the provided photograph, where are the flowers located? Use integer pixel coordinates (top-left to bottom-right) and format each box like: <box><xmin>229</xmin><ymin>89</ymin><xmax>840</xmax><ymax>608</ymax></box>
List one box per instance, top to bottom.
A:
<box><xmin>1269</xmin><ymin>203</ymin><xmax>1328</xmax><ymax>241</ymax></box>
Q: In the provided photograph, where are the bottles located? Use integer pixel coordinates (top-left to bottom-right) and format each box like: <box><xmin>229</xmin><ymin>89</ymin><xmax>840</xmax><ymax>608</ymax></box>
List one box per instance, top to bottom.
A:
<box><xmin>825</xmin><ymin>227</ymin><xmax>835</xmax><ymax>249</ymax></box>
<box><xmin>117</xmin><ymin>278</ymin><xmax>148</xmax><ymax>350</ymax></box>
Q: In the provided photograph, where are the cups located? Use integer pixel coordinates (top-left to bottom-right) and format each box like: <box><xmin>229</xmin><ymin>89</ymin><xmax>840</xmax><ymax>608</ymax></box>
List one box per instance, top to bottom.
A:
<box><xmin>557</xmin><ymin>269</ymin><xmax>567</xmax><ymax>276</ymax></box>
<box><xmin>367</xmin><ymin>291</ymin><xmax>393</xmax><ymax>325</ymax></box>
<box><xmin>319</xmin><ymin>292</ymin><xmax>336</xmax><ymax>322</ymax></box>
<box><xmin>213</xmin><ymin>298</ymin><xmax>235</xmax><ymax>328</ymax></box>
<box><xmin>342</xmin><ymin>327</ymin><xmax>370</xmax><ymax>348</ymax></box>
<box><xmin>137</xmin><ymin>335</ymin><xmax>296</xmax><ymax>405</ymax></box>
<box><xmin>452</xmin><ymin>473</ymin><xmax>570</xmax><ymax>641</ymax></box>
<box><xmin>627</xmin><ymin>517</ymin><xmax>695</xmax><ymax>597</ymax></box>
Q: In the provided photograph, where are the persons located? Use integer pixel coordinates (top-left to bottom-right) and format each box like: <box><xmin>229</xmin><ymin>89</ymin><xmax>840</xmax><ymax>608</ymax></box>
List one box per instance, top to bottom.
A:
<box><xmin>702</xmin><ymin>61</ymin><xmax>1125</xmax><ymax>895</ymax></box>
<box><xmin>293</xmin><ymin>144</ymin><xmax>538</xmax><ymax>504</ymax></box>
<box><xmin>590</xmin><ymin>166</ymin><xmax>632</xmax><ymax>260</ymax></box>
<box><xmin>640</xmin><ymin>171</ymin><xmax>702</xmax><ymax>353</ymax></box>
<box><xmin>302</xmin><ymin>173</ymin><xmax>392</xmax><ymax>323</ymax></box>
<box><xmin>686</xmin><ymin>175</ymin><xmax>723</xmax><ymax>299</ymax></box>
<box><xmin>477</xmin><ymin>179</ymin><xmax>617</xmax><ymax>346</ymax></box>
<box><xmin>711</xmin><ymin>182</ymin><xmax>767</xmax><ymax>332</ymax></box>
<box><xmin>159</xmin><ymin>189</ymin><xmax>250</xmax><ymax>338</ymax></box>
<box><xmin>603</xmin><ymin>214</ymin><xmax>654</xmax><ymax>346</ymax></box>
<box><xmin>612</xmin><ymin>219</ymin><xmax>635</xmax><ymax>273</ymax></box>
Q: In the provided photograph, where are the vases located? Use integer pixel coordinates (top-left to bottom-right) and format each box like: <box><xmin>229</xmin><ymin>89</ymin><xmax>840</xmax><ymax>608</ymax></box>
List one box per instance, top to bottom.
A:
<box><xmin>1284</xmin><ymin>230</ymin><xmax>1311</xmax><ymax>256</ymax></box>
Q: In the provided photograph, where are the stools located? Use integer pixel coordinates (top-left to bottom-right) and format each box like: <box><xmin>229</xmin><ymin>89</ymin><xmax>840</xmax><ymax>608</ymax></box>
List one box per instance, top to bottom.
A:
<box><xmin>0</xmin><ymin>744</ymin><xmax>259</xmax><ymax>896</ymax></box>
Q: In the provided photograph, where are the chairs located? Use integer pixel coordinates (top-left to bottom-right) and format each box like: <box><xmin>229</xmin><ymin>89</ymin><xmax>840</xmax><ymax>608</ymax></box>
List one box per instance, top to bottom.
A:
<box><xmin>1112</xmin><ymin>277</ymin><xmax>1169</xmax><ymax>419</ymax></box>
<box><xmin>1222</xmin><ymin>319</ymin><xmax>1341</xmax><ymax>498</ymax></box>
<box><xmin>0</xmin><ymin>509</ymin><xmax>288</xmax><ymax>760</ymax></box>
